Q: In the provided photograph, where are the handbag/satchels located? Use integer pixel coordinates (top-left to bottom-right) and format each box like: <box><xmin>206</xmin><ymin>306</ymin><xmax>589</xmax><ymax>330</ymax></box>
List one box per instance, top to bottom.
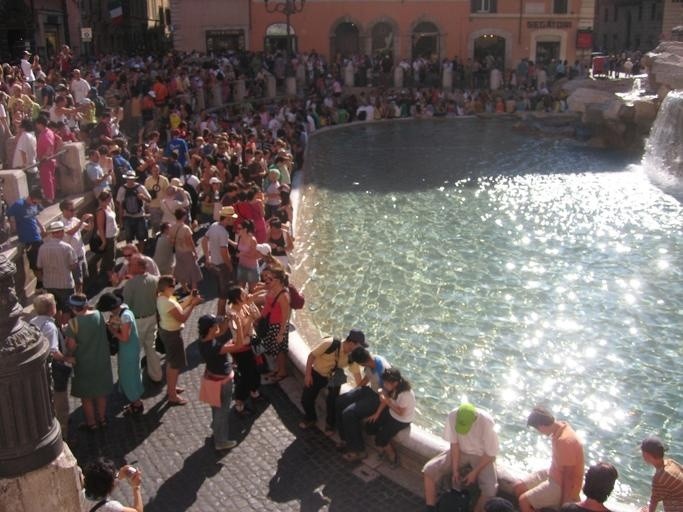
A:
<box><xmin>90</xmin><ymin>232</ymin><xmax>103</xmax><ymax>253</ymax></box>
<box><xmin>253</xmin><ymin>315</ymin><xmax>269</xmax><ymax>336</ymax></box>
<box><xmin>155</xmin><ymin>331</ymin><xmax>165</xmax><ymax>354</ymax></box>
<box><xmin>107</xmin><ymin>337</ymin><xmax>119</xmax><ymax>355</ymax></box>
<box><xmin>250</xmin><ymin>343</ymin><xmax>273</xmax><ymax>373</ymax></box>
<box><xmin>327</xmin><ymin>368</ymin><xmax>347</xmax><ymax>388</ymax></box>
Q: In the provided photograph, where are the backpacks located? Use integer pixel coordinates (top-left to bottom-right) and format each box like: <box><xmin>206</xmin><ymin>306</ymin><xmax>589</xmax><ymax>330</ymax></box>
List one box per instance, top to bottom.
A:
<box><xmin>122</xmin><ymin>184</ymin><xmax>143</xmax><ymax>214</ymax></box>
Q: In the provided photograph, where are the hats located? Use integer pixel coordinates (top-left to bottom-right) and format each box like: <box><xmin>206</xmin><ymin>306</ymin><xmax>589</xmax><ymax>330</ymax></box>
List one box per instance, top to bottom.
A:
<box><xmin>122</xmin><ymin>170</ymin><xmax>140</xmax><ymax>179</ymax></box>
<box><xmin>209</xmin><ymin>177</ymin><xmax>222</xmax><ymax>184</ymax></box>
<box><xmin>219</xmin><ymin>206</ymin><xmax>238</xmax><ymax>218</ymax></box>
<box><xmin>48</xmin><ymin>221</ymin><xmax>67</xmax><ymax>233</ymax></box>
<box><xmin>256</xmin><ymin>242</ymin><xmax>272</xmax><ymax>256</ymax></box>
<box><xmin>98</xmin><ymin>292</ymin><xmax>122</xmax><ymax>309</ymax></box>
<box><xmin>197</xmin><ymin>314</ymin><xmax>224</xmax><ymax>329</ymax></box>
<box><xmin>349</xmin><ymin>328</ymin><xmax>368</xmax><ymax>348</ymax></box>
<box><xmin>455</xmin><ymin>403</ymin><xmax>477</xmax><ymax>434</ymax></box>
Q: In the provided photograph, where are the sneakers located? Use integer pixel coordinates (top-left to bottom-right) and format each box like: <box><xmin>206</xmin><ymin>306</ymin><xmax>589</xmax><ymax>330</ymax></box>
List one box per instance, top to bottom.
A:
<box><xmin>250</xmin><ymin>389</ymin><xmax>260</xmax><ymax>399</ymax></box>
<box><xmin>235</xmin><ymin>400</ymin><xmax>245</xmax><ymax>412</ymax></box>
<box><xmin>297</xmin><ymin>421</ymin><xmax>398</xmax><ymax>469</ymax></box>
<box><xmin>215</xmin><ymin>440</ymin><xmax>237</xmax><ymax>450</ymax></box>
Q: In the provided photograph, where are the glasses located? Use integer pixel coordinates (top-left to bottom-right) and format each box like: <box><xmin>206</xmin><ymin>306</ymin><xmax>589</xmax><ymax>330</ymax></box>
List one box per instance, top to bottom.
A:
<box><xmin>32</xmin><ymin>200</ymin><xmax>38</xmax><ymax>205</ymax></box>
<box><xmin>124</xmin><ymin>253</ymin><xmax>132</xmax><ymax>257</ymax></box>
<box><xmin>267</xmin><ymin>275</ymin><xmax>278</xmax><ymax>282</ymax></box>
<box><xmin>169</xmin><ymin>284</ymin><xmax>176</xmax><ymax>288</ymax></box>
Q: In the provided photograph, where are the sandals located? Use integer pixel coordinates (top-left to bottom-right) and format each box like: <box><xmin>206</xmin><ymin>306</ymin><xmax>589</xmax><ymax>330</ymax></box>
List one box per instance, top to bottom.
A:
<box><xmin>78</xmin><ymin>386</ymin><xmax>188</xmax><ymax>433</ymax></box>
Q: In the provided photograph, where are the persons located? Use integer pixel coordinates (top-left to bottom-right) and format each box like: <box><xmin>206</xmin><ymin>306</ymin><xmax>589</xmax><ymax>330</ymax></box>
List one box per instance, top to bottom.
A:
<box><xmin>1</xmin><ymin>45</ymin><xmax>308</xmax><ymax>450</ymax></box>
<box><xmin>310</xmin><ymin>52</ymin><xmax>588</xmax><ymax>129</ymax></box>
<box><xmin>605</xmin><ymin>53</ymin><xmax>646</xmax><ymax>79</ymax></box>
<box><xmin>300</xmin><ymin>328</ymin><xmax>370</xmax><ymax>436</ymax></box>
<box><xmin>335</xmin><ymin>346</ymin><xmax>384</xmax><ymax>460</ymax></box>
<box><xmin>368</xmin><ymin>368</ymin><xmax>417</xmax><ymax>468</ymax></box>
<box><xmin>512</xmin><ymin>405</ymin><xmax>584</xmax><ymax>512</ymax></box>
<box><xmin>420</xmin><ymin>406</ymin><xmax>499</xmax><ymax>512</ymax></box>
<box><xmin>641</xmin><ymin>439</ymin><xmax>683</xmax><ymax>511</ymax></box>
<box><xmin>78</xmin><ymin>462</ymin><xmax>143</xmax><ymax>512</ymax></box>
<box><xmin>561</xmin><ymin>462</ymin><xmax>618</xmax><ymax>512</ymax></box>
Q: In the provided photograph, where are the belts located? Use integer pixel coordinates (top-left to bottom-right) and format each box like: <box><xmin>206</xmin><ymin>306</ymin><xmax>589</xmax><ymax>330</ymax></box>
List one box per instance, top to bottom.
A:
<box><xmin>135</xmin><ymin>314</ymin><xmax>152</xmax><ymax>319</ymax></box>
